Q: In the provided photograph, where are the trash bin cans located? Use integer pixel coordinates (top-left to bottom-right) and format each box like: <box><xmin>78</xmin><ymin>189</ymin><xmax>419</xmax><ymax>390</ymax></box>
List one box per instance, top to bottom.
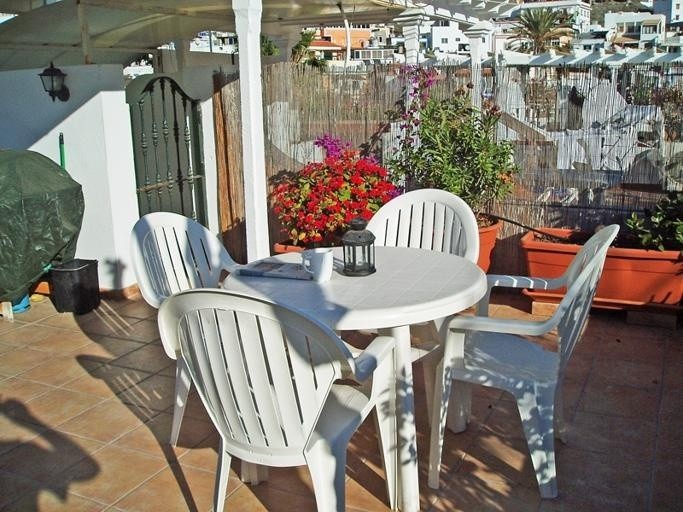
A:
<box><xmin>48</xmin><ymin>258</ymin><xmax>99</xmax><ymax>315</ymax></box>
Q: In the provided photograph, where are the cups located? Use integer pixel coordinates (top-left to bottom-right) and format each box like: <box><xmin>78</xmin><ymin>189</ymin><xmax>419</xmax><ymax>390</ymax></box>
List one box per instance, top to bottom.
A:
<box><xmin>302</xmin><ymin>248</ymin><xmax>334</xmax><ymax>283</ymax></box>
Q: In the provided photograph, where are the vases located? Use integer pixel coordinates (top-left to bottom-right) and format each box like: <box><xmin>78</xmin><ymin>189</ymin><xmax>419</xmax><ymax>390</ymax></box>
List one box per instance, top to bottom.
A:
<box><xmin>469</xmin><ymin>208</ymin><xmax>504</xmax><ymax>274</ymax></box>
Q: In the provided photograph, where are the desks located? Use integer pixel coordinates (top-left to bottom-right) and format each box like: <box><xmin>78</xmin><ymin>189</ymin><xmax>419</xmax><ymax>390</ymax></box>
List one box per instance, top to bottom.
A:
<box><xmin>219</xmin><ymin>239</ymin><xmax>489</xmax><ymax>512</ymax></box>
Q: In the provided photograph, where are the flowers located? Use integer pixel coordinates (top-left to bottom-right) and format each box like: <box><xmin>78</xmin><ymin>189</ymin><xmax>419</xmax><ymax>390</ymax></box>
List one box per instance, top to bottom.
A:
<box><xmin>383</xmin><ymin>62</ymin><xmax>525</xmax><ymax>228</ymax></box>
<box><xmin>269</xmin><ymin>132</ymin><xmax>397</xmax><ymax>247</ymax></box>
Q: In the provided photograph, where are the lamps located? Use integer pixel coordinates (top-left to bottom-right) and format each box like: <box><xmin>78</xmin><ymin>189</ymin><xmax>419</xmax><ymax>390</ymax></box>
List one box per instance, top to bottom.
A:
<box><xmin>34</xmin><ymin>57</ymin><xmax>70</xmax><ymax>102</ymax></box>
<box><xmin>341</xmin><ymin>218</ymin><xmax>377</xmax><ymax>277</ymax></box>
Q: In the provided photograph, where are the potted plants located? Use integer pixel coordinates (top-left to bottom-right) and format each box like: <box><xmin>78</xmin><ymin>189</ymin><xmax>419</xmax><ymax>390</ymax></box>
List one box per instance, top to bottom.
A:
<box><xmin>521</xmin><ymin>199</ymin><xmax>682</xmax><ymax>319</ymax></box>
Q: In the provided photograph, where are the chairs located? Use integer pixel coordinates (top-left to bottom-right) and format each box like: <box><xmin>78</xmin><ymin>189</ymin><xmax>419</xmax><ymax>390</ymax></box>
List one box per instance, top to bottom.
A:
<box><xmin>363</xmin><ymin>190</ymin><xmax>478</xmax><ymax>435</ymax></box>
<box><xmin>124</xmin><ymin>209</ymin><xmax>242</xmax><ymax>447</ymax></box>
<box><xmin>424</xmin><ymin>218</ymin><xmax>619</xmax><ymax>501</ymax></box>
<box><xmin>151</xmin><ymin>287</ymin><xmax>409</xmax><ymax>512</ymax></box>
